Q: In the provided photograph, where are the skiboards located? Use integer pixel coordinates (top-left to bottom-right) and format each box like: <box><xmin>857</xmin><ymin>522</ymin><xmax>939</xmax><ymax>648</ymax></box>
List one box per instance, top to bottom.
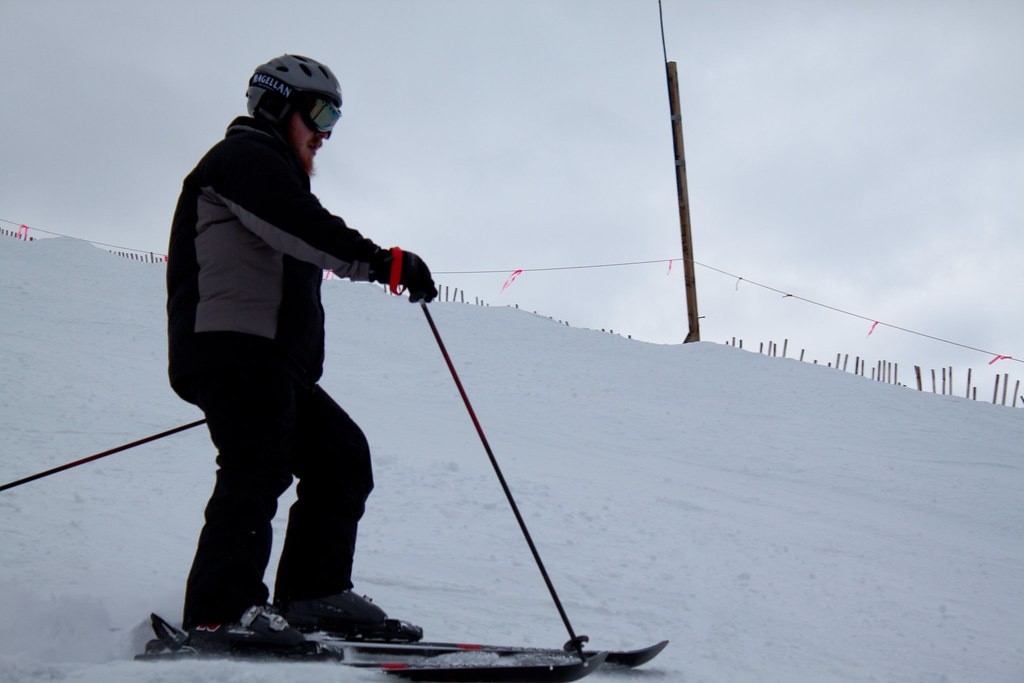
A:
<box><xmin>131</xmin><ymin>610</ymin><xmax>672</xmax><ymax>683</ymax></box>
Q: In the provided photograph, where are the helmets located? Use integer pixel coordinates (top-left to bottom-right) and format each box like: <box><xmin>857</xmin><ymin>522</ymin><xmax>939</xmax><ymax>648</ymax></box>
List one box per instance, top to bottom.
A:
<box><xmin>246</xmin><ymin>54</ymin><xmax>343</xmax><ymax>128</ymax></box>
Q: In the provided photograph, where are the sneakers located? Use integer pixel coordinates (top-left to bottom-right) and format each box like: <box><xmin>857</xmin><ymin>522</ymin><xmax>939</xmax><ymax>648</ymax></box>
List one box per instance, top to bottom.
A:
<box><xmin>302</xmin><ymin>589</ymin><xmax>423</xmax><ymax>641</ymax></box>
<box><xmin>188</xmin><ymin>605</ymin><xmax>306</xmax><ymax>649</ymax></box>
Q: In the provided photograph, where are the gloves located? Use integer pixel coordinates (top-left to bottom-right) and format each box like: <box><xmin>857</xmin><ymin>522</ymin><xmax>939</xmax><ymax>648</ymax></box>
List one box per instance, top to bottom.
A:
<box><xmin>391</xmin><ymin>246</ymin><xmax>438</xmax><ymax>303</ymax></box>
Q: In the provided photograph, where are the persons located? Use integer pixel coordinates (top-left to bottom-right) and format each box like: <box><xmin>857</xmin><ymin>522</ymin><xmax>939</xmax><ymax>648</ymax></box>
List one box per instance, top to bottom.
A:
<box><xmin>166</xmin><ymin>55</ymin><xmax>438</xmax><ymax>655</ymax></box>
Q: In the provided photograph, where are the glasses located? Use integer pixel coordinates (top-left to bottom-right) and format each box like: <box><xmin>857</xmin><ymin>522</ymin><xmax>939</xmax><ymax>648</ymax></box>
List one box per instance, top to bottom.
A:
<box><xmin>301</xmin><ymin>96</ymin><xmax>342</xmax><ymax>139</ymax></box>
